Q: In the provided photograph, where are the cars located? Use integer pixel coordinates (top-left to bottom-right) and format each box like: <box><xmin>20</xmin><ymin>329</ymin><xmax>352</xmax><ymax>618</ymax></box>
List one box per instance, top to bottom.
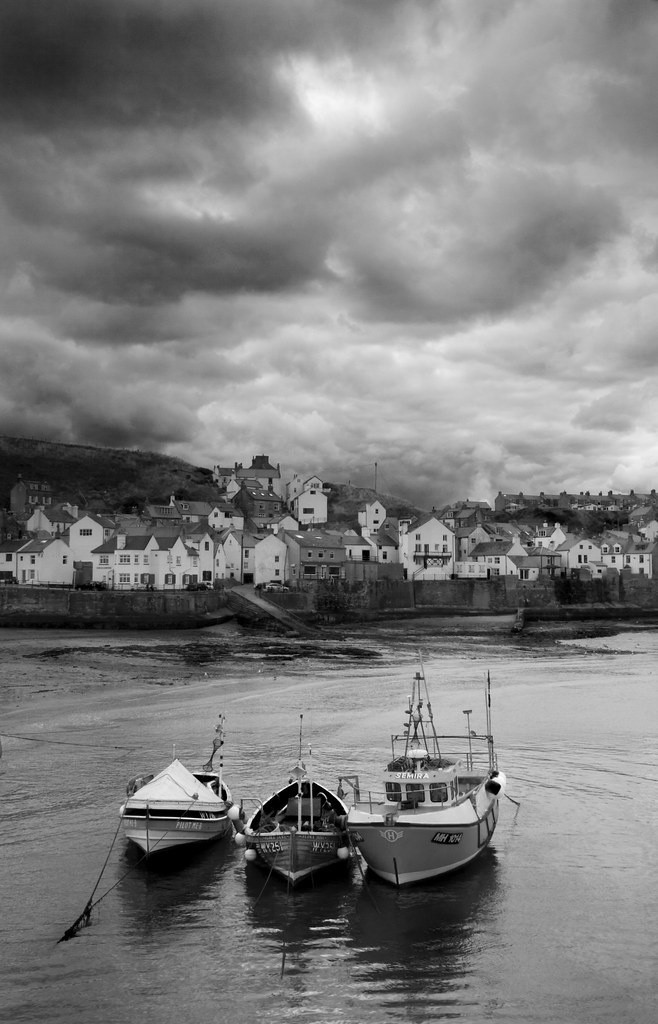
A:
<box><xmin>5</xmin><ymin>577</ymin><xmax>19</xmax><ymax>584</ymax></box>
<box><xmin>85</xmin><ymin>581</ymin><xmax>106</xmax><ymax>591</ymax></box>
<box><xmin>203</xmin><ymin>581</ymin><xmax>214</xmax><ymax>590</ymax></box>
<box><xmin>253</xmin><ymin>581</ymin><xmax>269</xmax><ymax>589</ymax></box>
<box><xmin>188</xmin><ymin>583</ymin><xmax>208</xmax><ymax>590</ymax></box>
<box><xmin>266</xmin><ymin>583</ymin><xmax>289</xmax><ymax>593</ymax></box>
<box><xmin>139</xmin><ymin>585</ymin><xmax>151</xmax><ymax>592</ymax></box>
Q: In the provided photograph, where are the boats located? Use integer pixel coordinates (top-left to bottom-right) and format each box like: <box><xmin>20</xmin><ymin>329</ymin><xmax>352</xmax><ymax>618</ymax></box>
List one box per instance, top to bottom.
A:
<box><xmin>338</xmin><ymin>649</ymin><xmax>507</xmax><ymax>887</ymax></box>
<box><xmin>240</xmin><ymin>714</ymin><xmax>353</xmax><ymax>887</ymax></box>
<box><xmin>120</xmin><ymin>715</ymin><xmax>233</xmax><ymax>856</ymax></box>
